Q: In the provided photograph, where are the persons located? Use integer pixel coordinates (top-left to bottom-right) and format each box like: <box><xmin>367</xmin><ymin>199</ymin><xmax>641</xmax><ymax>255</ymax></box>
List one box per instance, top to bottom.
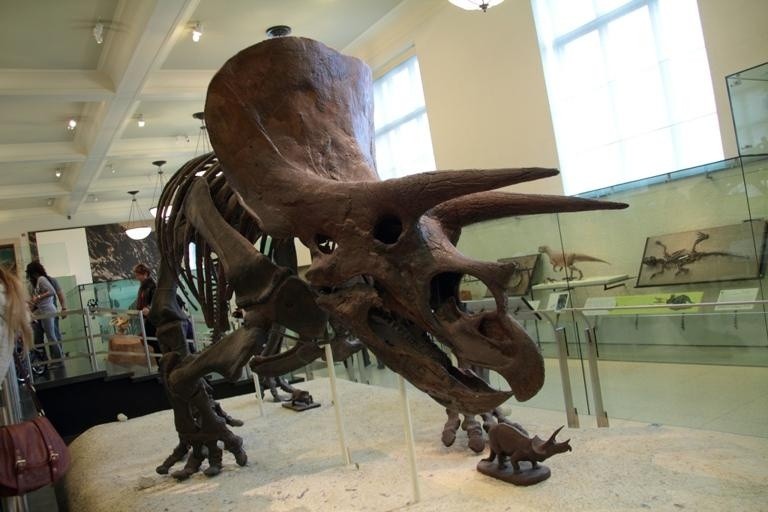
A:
<box><xmin>25</xmin><ymin>260</ymin><xmax>67</xmax><ymax>371</ymax></box>
<box><xmin>0</xmin><ymin>262</ymin><xmax>36</xmax><ymax>512</ymax></box>
<box><xmin>134</xmin><ymin>263</ymin><xmax>162</xmax><ymax>373</ymax></box>
<box><xmin>176</xmin><ymin>294</ymin><xmax>196</xmax><ymax>354</ymax></box>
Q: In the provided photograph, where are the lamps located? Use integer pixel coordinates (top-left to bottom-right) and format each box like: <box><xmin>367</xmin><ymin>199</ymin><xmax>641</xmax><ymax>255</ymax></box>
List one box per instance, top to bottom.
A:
<box><xmin>45</xmin><ymin>0</ymin><xmax>206</xmax><ymax>239</ymax></box>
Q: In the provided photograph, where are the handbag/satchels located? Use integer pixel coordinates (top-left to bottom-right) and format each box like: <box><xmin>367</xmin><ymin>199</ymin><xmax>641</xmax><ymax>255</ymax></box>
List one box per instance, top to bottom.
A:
<box><xmin>0</xmin><ymin>412</ymin><xmax>72</xmax><ymax>498</ymax></box>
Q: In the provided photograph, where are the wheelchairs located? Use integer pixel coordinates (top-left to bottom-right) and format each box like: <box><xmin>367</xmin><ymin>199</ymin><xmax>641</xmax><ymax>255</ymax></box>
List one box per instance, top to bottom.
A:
<box><xmin>14</xmin><ymin>306</ymin><xmax>48</xmax><ymax>382</ymax></box>
